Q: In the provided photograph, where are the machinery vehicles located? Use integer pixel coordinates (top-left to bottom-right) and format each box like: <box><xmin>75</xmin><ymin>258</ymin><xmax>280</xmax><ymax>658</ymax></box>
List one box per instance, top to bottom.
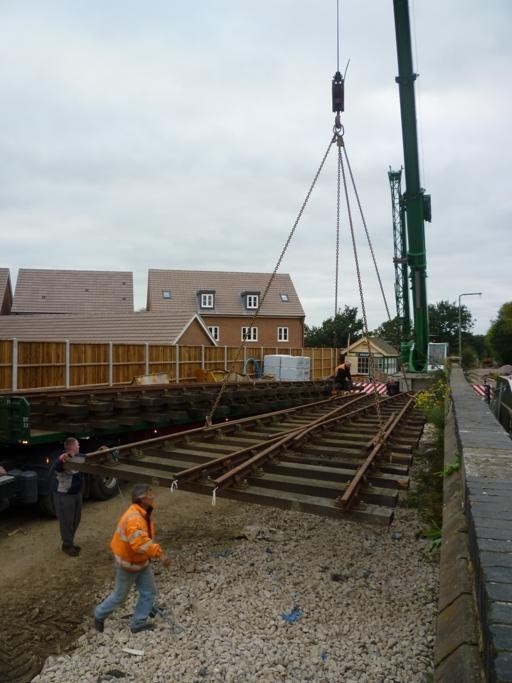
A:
<box><xmin>331</xmin><ymin>0</ymin><xmax>450</xmax><ymax>374</ymax></box>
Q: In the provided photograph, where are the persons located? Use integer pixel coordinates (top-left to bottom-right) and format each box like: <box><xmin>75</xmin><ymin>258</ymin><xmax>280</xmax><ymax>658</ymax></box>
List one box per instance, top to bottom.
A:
<box><xmin>335</xmin><ymin>360</ymin><xmax>353</xmax><ymax>391</ymax></box>
<box><xmin>47</xmin><ymin>438</ymin><xmax>109</xmax><ymax>555</ymax></box>
<box><xmin>94</xmin><ymin>486</ymin><xmax>171</xmax><ymax>633</ymax></box>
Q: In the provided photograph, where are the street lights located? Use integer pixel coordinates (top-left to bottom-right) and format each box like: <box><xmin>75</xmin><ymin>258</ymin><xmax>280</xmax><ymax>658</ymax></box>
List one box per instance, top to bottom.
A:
<box><xmin>459</xmin><ymin>293</ymin><xmax>482</xmax><ymax>365</ymax></box>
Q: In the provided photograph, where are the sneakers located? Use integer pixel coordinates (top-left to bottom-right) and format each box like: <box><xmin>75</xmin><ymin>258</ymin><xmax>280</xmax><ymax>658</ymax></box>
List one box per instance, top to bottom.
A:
<box><xmin>61</xmin><ymin>543</ymin><xmax>82</xmax><ymax>556</ymax></box>
<box><xmin>94</xmin><ymin>617</ymin><xmax>105</xmax><ymax>633</ymax></box>
<box><xmin>131</xmin><ymin>622</ymin><xmax>158</xmax><ymax>634</ymax></box>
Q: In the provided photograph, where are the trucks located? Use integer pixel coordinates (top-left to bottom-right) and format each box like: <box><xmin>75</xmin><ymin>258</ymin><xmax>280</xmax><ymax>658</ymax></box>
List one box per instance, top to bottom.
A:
<box><xmin>0</xmin><ymin>387</ymin><xmax>238</xmax><ymax>515</ymax></box>
